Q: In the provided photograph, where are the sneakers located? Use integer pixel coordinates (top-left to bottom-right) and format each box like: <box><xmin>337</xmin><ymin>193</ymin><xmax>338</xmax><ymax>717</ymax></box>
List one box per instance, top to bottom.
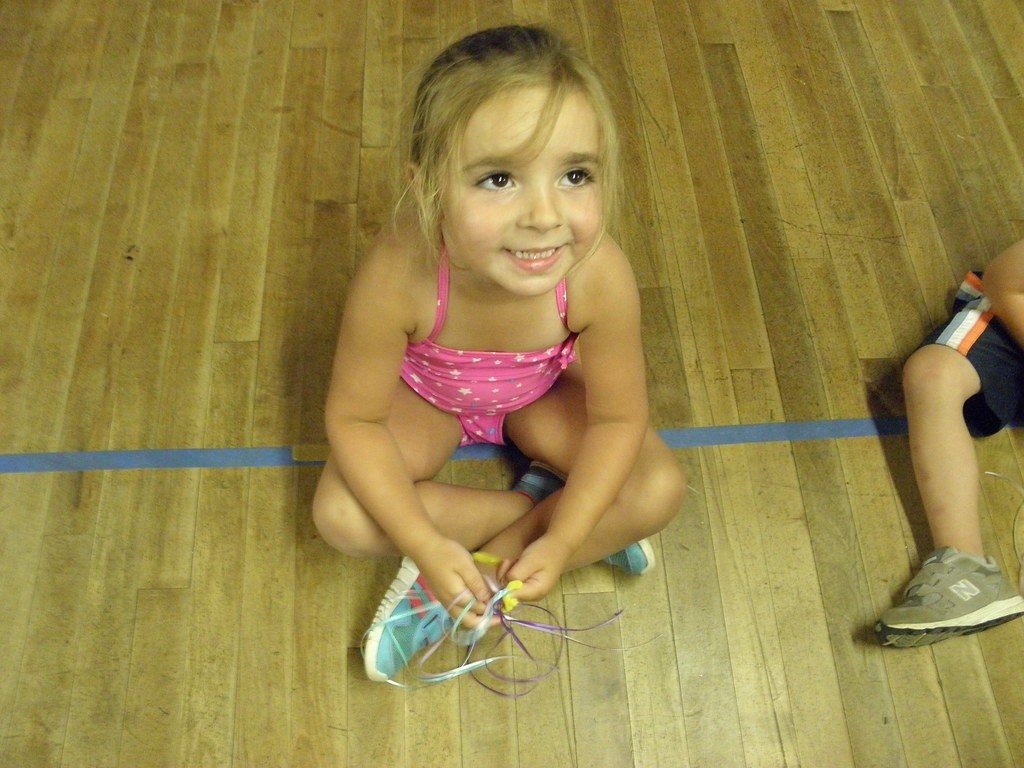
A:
<box><xmin>510</xmin><ymin>458</ymin><xmax>656</xmax><ymax>576</ymax></box>
<box><xmin>872</xmin><ymin>546</ymin><xmax>1023</xmax><ymax>649</ymax></box>
<box><xmin>363</xmin><ymin>552</ymin><xmax>502</xmax><ymax>683</ymax></box>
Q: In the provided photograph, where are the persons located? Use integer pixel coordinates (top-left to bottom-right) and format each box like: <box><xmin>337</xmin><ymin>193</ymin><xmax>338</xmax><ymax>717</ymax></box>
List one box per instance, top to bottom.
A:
<box><xmin>310</xmin><ymin>23</ymin><xmax>686</xmax><ymax>684</ymax></box>
<box><xmin>872</xmin><ymin>237</ymin><xmax>1024</xmax><ymax>649</ymax></box>
